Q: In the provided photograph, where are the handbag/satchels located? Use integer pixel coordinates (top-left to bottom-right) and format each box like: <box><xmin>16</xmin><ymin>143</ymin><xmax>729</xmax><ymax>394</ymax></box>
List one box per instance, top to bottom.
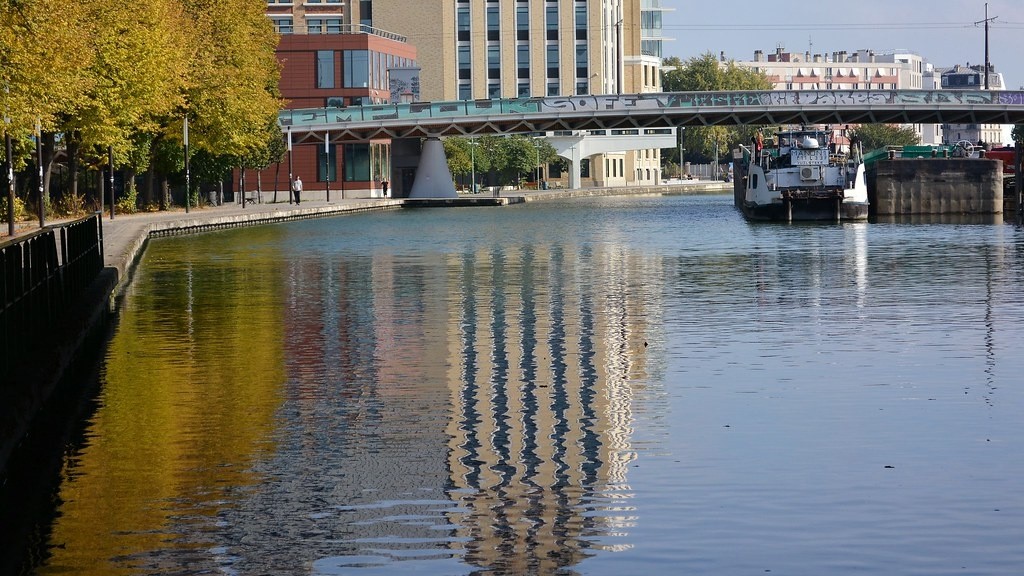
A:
<box><xmin>292</xmin><ymin>182</ymin><xmax>295</xmax><ymax>191</ymax></box>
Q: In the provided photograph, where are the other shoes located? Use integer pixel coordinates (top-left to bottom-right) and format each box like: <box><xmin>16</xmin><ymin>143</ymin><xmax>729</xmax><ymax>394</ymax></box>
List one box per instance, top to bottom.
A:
<box><xmin>298</xmin><ymin>203</ymin><xmax>300</xmax><ymax>205</ymax></box>
<box><xmin>295</xmin><ymin>204</ymin><xmax>297</xmax><ymax>206</ymax></box>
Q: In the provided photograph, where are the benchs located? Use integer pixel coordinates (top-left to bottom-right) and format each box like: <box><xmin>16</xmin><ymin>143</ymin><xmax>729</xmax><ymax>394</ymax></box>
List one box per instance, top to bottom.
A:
<box><xmin>555</xmin><ymin>182</ymin><xmax>564</xmax><ymax>189</ymax></box>
<box><xmin>478</xmin><ymin>184</ymin><xmax>489</xmax><ymax>193</ymax></box>
<box><xmin>245</xmin><ymin>198</ymin><xmax>257</xmax><ymax>204</ymax></box>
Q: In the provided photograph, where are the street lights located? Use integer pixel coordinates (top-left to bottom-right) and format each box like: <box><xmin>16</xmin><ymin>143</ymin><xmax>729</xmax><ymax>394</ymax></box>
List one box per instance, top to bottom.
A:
<box><xmin>680</xmin><ymin>127</ymin><xmax>686</xmax><ymax>146</ymax></box>
<box><xmin>467</xmin><ymin>134</ymin><xmax>479</xmax><ymax>193</ymax></box>
<box><xmin>534</xmin><ymin>140</ymin><xmax>542</xmax><ymax>191</ymax></box>
<box><xmin>569</xmin><ymin>143</ymin><xmax>576</xmax><ymax>188</ymax></box>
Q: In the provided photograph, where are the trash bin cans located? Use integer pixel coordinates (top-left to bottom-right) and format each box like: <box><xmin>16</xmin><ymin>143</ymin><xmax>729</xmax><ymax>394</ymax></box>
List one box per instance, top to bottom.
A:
<box><xmin>543</xmin><ymin>182</ymin><xmax>549</xmax><ymax>190</ymax></box>
<box><xmin>472</xmin><ymin>183</ymin><xmax>479</xmax><ymax>194</ymax></box>
<box><xmin>209</xmin><ymin>191</ymin><xmax>217</xmax><ymax>207</ymax></box>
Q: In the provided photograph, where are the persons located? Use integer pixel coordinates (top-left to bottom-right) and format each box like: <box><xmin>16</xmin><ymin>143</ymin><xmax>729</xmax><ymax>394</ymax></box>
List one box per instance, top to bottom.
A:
<box><xmin>751</xmin><ymin>127</ymin><xmax>763</xmax><ymax>152</ymax></box>
<box><xmin>682</xmin><ymin>172</ymin><xmax>693</xmax><ymax>180</ymax></box>
<box><xmin>292</xmin><ymin>176</ymin><xmax>302</xmax><ymax>205</ymax></box>
<box><xmin>724</xmin><ymin>177</ymin><xmax>730</xmax><ymax>182</ymax></box>
<box><xmin>381</xmin><ymin>178</ymin><xmax>388</xmax><ymax>197</ymax></box>
<box><xmin>511</xmin><ymin>180</ymin><xmax>515</xmax><ymax>190</ymax></box>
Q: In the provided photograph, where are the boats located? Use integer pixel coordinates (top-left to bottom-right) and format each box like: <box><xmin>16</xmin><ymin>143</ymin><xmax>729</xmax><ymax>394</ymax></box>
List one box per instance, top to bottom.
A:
<box><xmin>733</xmin><ymin>125</ymin><xmax>846</xmax><ymax>222</ymax></box>
